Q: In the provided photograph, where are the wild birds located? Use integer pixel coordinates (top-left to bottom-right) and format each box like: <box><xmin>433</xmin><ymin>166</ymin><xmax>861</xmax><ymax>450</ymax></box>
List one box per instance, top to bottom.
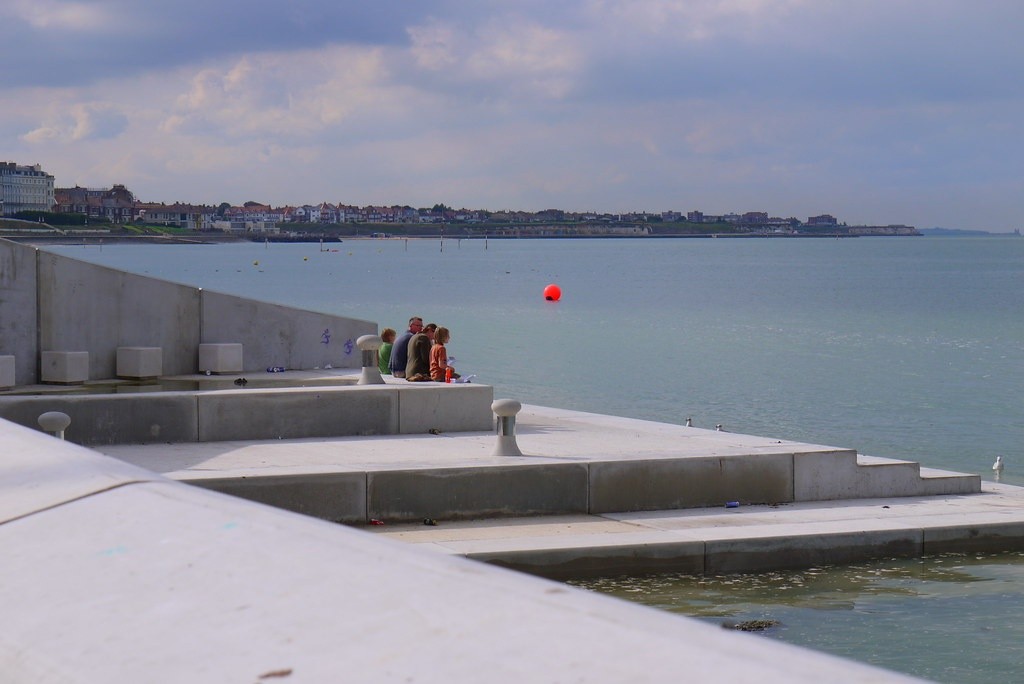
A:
<box><xmin>684</xmin><ymin>417</ymin><xmax>695</xmax><ymax>427</ymax></box>
<box><xmin>715</xmin><ymin>423</ymin><xmax>731</xmax><ymax>433</ymax></box>
<box><xmin>992</xmin><ymin>455</ymin><xmax>1006</xmax><ymax>473</ymax></box>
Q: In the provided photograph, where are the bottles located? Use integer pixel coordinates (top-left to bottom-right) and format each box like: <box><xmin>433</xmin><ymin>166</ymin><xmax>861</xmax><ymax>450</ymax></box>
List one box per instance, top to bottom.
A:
<box><xmin>445</xmin><ymin>366</ymin><xmax>450</xmax><ymax>383</ymax></box>
<box><xmin>266</xmin><ymin>367</ymin><xmax>286</xmax><ymax>372</ymax></box>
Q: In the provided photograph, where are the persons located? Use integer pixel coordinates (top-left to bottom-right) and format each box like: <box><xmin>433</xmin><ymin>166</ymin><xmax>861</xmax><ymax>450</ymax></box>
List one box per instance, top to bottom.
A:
<box><xmin>388</xmin><ymin>317</ymin><xmax>424</xmax><ymax>378</ymax></box>
<box><xmin>405</xmin><ymin>324</ymin><xmax>438</xmax><ymax>382</ymax></box>
<box><xmin>430</xmin><ymin>327</ymin><xmax>461</xmax><ymax>383</ymax></box>
<box><xmin>378</xmin><ymin>329</ymin><xmax>396</xmax><ymax>375</ymax></box>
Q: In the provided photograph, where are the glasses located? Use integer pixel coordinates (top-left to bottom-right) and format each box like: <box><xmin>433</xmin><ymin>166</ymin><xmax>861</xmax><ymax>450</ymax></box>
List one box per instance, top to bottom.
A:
<box><xmin>413</xmin><ymin>323</ymin><xmax>424</xmax><ymax>328</ymax></box>
<box><xmin>429</xmin><ymin>328</ymin><xmax>435</xmax><ymax>333</ymax></box>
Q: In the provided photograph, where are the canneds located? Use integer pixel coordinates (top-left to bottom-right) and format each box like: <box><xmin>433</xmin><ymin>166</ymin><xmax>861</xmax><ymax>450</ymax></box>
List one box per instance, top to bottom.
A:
<box><xmin>266</xmin><ymin>367</ymin><xmax>277</xmax><ymax>372</ymax></box>
<box><xmin>277</xmin><ymin>367</ymin><xmax>285</xmax><ymax>372</ymax></box>
<box><xmin>429</xmin><ymin>428</ymin><xmax>438</xmax><ymax>434</ymax></box>
<box><xmin>726</xmin><ymin>502</ymin><xmax>739</xmax><ymax>507</ymax></box>
<box><xmin>424</xmin><ymin>519</ymin><xmax>436</xmax><ymax>525</ymax></box>
